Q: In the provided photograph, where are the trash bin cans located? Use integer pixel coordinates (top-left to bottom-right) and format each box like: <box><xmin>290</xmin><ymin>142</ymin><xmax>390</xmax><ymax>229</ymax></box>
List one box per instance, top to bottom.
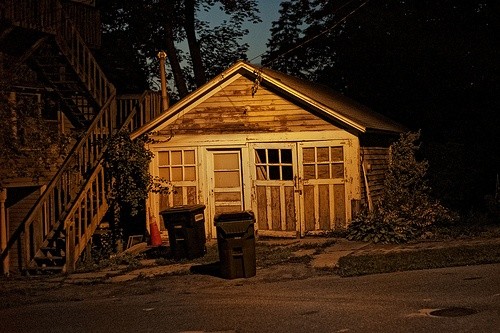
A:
<box><xmin>159</xmin><ymin>203</ymin><xmax>207</xmax><ymax>259</ymax></box>
<box><xmin>213</xmin><ymin>209</ymin><xmax>257</xmax><ymax>278</ymax></box>
